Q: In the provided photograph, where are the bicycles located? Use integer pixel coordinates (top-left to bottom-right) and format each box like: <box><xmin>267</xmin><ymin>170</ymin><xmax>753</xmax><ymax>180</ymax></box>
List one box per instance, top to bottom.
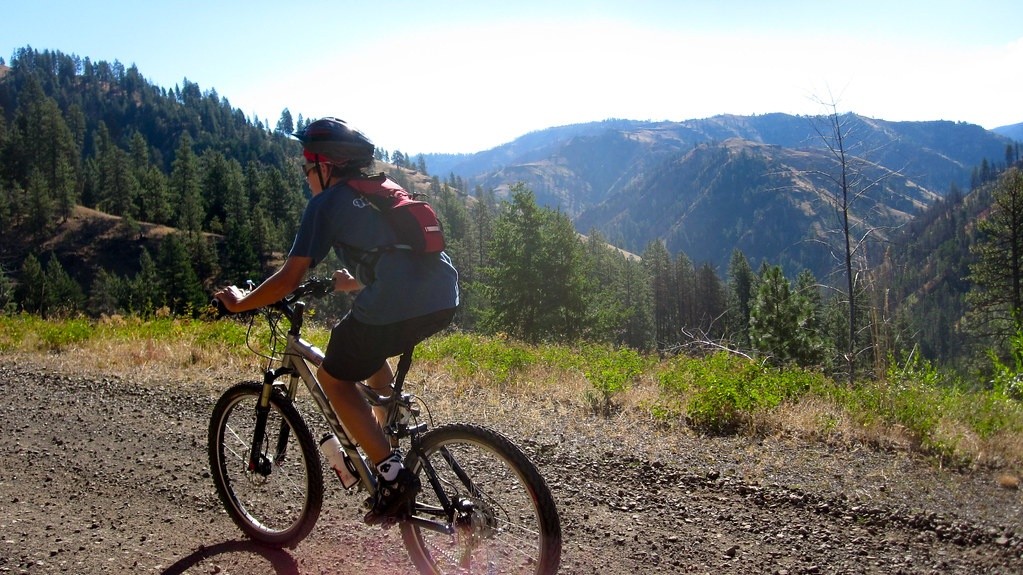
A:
<box><xmin>207</xmin><ymin>278</ymin><xmax>562</xmax><ymax>574</ymax></box>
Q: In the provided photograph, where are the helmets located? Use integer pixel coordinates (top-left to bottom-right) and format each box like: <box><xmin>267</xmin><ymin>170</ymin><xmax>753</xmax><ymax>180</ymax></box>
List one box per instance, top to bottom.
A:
<box><xmin>291</xmin><ymin>117</ymin><xmax>375</xmax><ymax>163</ymax></box>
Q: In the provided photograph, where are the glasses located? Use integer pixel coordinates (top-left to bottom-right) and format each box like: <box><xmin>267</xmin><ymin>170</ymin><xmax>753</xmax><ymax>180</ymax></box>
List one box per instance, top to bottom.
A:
<box><xmin>302</xmin><ymin>161</ymin><xmax>331</xmax><ymax>176</ymax></box>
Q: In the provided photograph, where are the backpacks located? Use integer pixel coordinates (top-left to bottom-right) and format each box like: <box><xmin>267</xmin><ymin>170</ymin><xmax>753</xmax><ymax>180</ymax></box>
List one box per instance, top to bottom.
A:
<box><xmin>342</xmin><ymin>172</ymin><xmax>446</xmax><ymax>256</ymax></box>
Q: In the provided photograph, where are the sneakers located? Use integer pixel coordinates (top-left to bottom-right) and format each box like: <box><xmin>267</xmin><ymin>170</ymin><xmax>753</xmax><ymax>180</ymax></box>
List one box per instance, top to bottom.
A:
<box><xmin>363</xmin><ymin>466</ymin><xmax>422</xmax><ymax>526</ymax></box>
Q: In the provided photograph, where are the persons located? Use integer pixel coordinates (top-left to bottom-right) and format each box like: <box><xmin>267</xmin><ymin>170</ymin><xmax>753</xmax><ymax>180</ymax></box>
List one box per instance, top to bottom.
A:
<box><xmin>213</xmin><ymin>117</ymin><xmax>460</xmax><ymax>528</ymax></box>
